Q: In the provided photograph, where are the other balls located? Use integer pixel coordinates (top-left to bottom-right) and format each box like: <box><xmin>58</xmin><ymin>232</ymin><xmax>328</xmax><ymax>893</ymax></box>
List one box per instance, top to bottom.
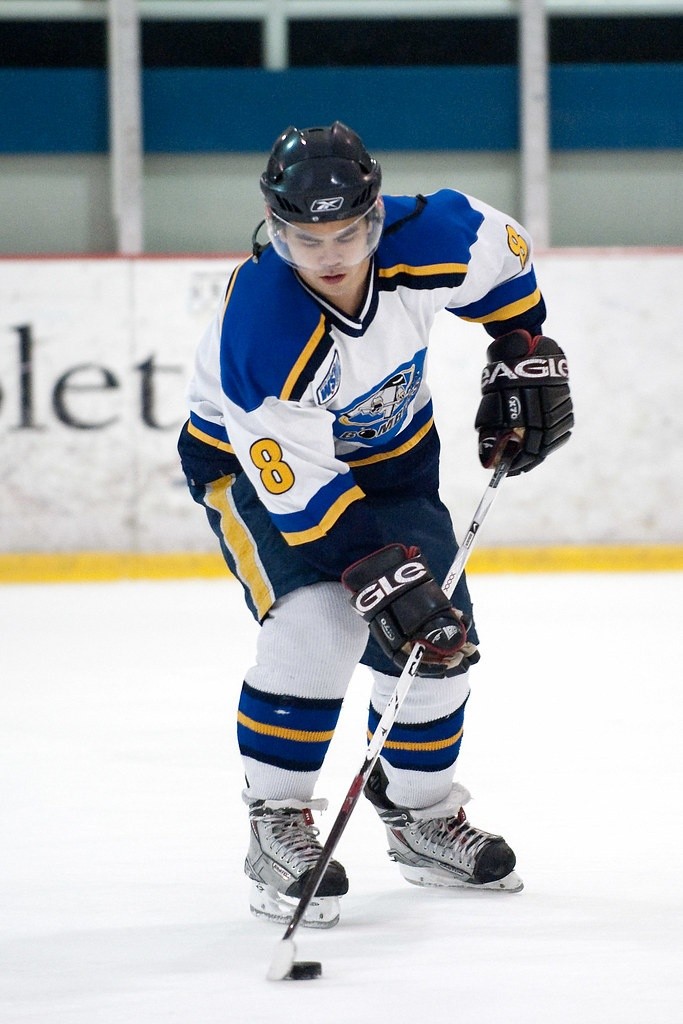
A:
<box><xmin>289</xmin><ymin>959</ymin><xmax>324</xmax><ymax>981</ymax></box>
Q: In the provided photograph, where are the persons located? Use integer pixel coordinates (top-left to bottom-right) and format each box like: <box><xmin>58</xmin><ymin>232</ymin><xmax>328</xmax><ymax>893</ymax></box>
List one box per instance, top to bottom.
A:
<box><xmin>177</xmin><ymin>123</ymin><xmax>574</xmax><ymax>929</ymax></box>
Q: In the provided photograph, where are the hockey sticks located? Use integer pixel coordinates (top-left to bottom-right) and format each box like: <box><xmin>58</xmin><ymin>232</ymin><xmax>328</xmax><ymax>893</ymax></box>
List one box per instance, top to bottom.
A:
<box><xmin>251</xmin><ymin>418</ymin><xmax>524</xmax><ymax>979</ymax></box>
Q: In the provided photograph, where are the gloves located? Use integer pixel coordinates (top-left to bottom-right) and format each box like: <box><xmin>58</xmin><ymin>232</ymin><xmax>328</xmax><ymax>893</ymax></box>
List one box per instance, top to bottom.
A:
<box><xmin>475</xmin><ymin>329</ymin><xmax>574</xmax><ymax>475</ymax></box>
<box><xmin>341</xmin><ymin>542</ymin><xmax>481</xmax><ymax>678</ymax></box>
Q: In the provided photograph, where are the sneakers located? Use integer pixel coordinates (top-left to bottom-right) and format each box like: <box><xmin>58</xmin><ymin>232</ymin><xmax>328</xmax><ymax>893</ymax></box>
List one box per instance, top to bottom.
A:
<box><xmin>363</xmin><ymin>758</ymin><xmax>524</xmax><ymax>892</ymax></box>
<box><xmin>241</xmin><ymin>789</ymin><xmax>349</xmax><ymax>927</ymax></box>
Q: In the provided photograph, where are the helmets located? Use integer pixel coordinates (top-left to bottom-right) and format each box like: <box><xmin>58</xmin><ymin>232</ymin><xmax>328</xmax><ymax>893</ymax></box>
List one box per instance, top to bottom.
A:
<box><xmin>259</xmin><ymin>120</ymin><xmax>381</xmax><ymax>223</ymax></box>
